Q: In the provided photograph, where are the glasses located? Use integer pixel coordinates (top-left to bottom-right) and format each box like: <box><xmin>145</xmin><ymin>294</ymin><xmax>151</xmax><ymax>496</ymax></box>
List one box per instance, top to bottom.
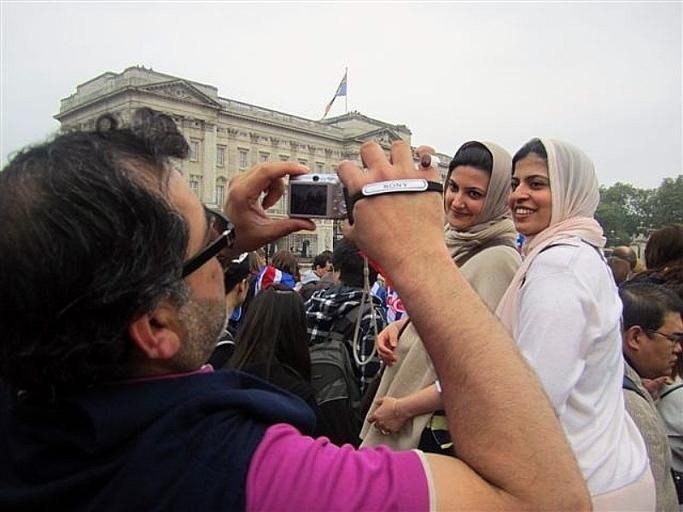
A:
<box><xmin>138</xmin><ymin>203</ymin><xmax>236</xmax><ymax>313</ymax></box>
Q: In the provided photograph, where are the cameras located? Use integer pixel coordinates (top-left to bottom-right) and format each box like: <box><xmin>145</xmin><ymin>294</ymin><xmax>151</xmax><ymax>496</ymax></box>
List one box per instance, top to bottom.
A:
<box><xmin>286</xmin><ymin>172</ymin><xmax>349</xmax><ymax>220</ymax></box>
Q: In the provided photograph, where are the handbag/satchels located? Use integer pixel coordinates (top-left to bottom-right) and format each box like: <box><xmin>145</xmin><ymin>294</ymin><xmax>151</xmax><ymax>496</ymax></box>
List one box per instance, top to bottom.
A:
<box><xmin>309</xmin><ymin>339</ymin><xmax>363</xmax><ymax>447</ymax></box>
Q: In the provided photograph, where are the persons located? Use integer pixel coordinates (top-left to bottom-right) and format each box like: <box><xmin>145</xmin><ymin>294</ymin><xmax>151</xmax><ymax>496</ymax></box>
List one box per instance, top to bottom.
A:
<box><xmin>1</xmin><ymin>106</ymin><xmax>597</xmax><ymax>512</ymax></box>
<box><xmin>208</xmin><ymin>136</ymin><xmax>683</xmax><ymax>512</ymax></box>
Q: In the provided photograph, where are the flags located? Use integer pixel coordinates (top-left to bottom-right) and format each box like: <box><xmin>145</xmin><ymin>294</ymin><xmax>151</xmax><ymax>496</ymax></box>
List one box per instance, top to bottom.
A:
<box><xmin>322</xmin><ymin>72</ymin><xmax>347</xmax><ymax>117</ymax></box>
